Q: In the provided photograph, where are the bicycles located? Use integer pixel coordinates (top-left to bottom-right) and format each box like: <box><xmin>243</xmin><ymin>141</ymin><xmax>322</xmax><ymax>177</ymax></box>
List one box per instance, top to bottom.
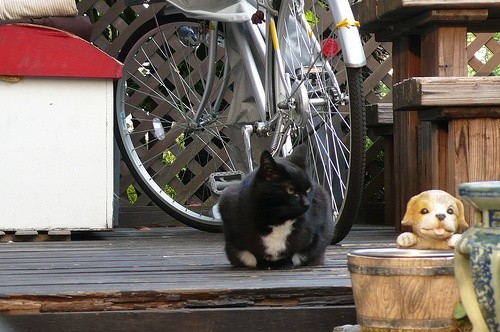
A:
<box><xmin>112</xmin><ymin>0</ymin><xmax>367</xmax><ymax>248</ymax></box>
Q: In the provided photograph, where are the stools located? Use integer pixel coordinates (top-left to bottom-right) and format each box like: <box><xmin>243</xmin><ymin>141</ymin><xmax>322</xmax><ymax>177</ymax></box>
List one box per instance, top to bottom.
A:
<box><xmin>394</xmin><ymin>76</ymin><xmax>500</xmax><ymax>234</ymax></box>
<box><xmin>364</xmin><ymin>103</ymin><xmax>394</xmax><ymax>225</ymax></box>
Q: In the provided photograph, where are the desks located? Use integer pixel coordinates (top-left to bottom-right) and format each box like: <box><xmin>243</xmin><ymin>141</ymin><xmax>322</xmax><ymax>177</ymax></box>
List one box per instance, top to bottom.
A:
<box><xmin>347</xmin><ymin>1</ymin><xmax>500</xmax><ymax>234</ymax></box>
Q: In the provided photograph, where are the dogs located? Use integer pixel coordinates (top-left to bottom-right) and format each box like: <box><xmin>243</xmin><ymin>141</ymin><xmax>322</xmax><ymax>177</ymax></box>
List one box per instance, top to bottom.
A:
<box><xmin>396</xmin><ymin>189</ymin><xmax>470</xmax><ymax>250</ymax></box>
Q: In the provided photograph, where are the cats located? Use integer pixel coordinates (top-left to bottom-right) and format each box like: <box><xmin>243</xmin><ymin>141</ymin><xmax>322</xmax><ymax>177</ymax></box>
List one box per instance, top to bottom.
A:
<box><xmin>218</xmin><ymin>143</ymin><xmax>334</xmax><ymax>270</ymax></box>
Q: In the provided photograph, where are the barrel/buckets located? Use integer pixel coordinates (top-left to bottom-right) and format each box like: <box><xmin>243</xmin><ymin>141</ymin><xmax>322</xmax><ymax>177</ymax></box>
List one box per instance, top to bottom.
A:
<box><xmin>348</xmin><ymin>249</ymin><xmax>463</xmax><ymax>332</ymax></box>
